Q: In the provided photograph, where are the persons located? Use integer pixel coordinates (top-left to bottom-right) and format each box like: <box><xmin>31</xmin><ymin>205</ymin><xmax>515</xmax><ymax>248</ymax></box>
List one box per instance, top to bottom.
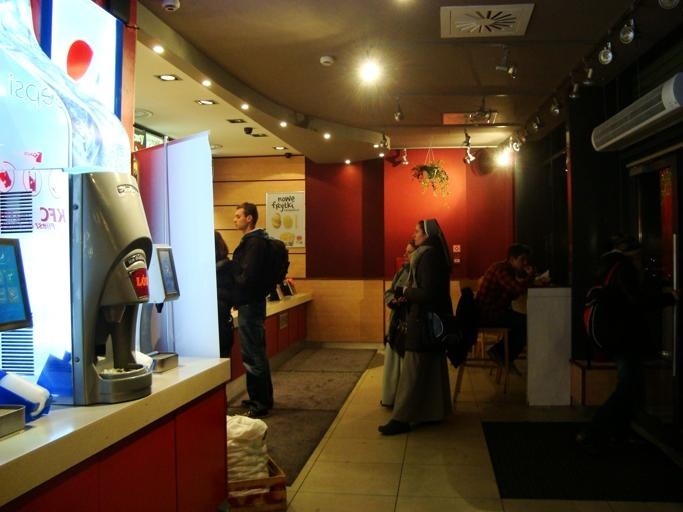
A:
<box><xmin>228</xmin><ymin>201</ymin><xmax>276</xmax><ymax>420</ymax></box>
<box><xmin>375</xmin><ymin>216</ymin><xmax>454</xmax><ymax>434</ymax></box>
<box><xmin>572</xmin><ymin>226</ymin><xmax>683</xmax><ymax>457</ymax></box>
<box><xmin>212</xmin><ymin>228</ymin><xmax>236</xmax><ymax>360</ymax></box>
<box><xmin>376</xmin><ymin>239</ymin><xmax>415</xmax><ymax>411</ymax></box>
<box><xmin>476</xmin><ymin>242</ymin><xmax>538</xmax><ymax>379</ymax></box>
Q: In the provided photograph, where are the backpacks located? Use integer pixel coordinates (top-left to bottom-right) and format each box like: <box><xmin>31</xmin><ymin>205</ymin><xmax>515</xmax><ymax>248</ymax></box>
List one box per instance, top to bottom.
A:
<box><xmin>243</xmin><ymin>233</ymin><xmax>290</xmax><ymax>289</ymax></box>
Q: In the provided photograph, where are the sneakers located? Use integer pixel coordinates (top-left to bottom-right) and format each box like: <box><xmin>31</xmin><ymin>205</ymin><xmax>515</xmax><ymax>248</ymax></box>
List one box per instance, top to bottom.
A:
<box><xmin>242</xmin><ymin>399</ymin><xmax>252</xmax><ymax>408</ymax></box>
<box><xmin>251</xmin><ymin>401</ymin><xmax>268</xmax><ymax>414</ymax></box>
<box><xmin>379</xmin><ymin>419</ymin><xmax>412</xmax><ymax>434</ymax></box>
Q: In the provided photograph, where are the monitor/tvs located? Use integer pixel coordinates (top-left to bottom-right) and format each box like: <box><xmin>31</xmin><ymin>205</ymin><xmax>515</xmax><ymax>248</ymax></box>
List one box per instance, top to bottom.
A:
<box><xmin>0</xmin><ymin>238</ymin><xmax>32</xmax><ymax>332</ymax></box>
<box><xmin>147</xmin><ymin>243</ymin><xmax>180</xmax><ymax>304</ymax></box>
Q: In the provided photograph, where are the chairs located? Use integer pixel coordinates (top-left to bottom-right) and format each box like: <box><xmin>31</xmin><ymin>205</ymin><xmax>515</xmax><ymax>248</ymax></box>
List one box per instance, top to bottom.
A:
<box><xmin>452</xmin><ymin>286</ymin><xmax>510</xmax><ymax>409</ymax></box>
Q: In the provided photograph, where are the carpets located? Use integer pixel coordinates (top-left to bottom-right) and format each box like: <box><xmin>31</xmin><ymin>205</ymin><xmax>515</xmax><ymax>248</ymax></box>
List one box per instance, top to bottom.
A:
<box><xmin>279</xmin><ymin>347</ymin><xmax>378</xmax><ymax>372</ymax></box>
<box><xmin>229</xmin><ymin>370</ymin><xmax>363</xmax><ymax>410</ymax></box>
<box><xmin>232</xmin><ymin>408</ymin><xmax>338</xmax><ymax>485</ymax></box>
<box><xmin>477</xmin><ymin>419</ymin><xmax>682</xmax><ymax>503</ymax></box>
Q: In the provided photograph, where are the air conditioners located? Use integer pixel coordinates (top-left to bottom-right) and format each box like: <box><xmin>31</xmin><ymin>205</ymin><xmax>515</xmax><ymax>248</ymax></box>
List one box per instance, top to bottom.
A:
<box><xmin>590</xmin><ymin>72</ymin><xmax>683</xmax><ymax>154</ymax></box>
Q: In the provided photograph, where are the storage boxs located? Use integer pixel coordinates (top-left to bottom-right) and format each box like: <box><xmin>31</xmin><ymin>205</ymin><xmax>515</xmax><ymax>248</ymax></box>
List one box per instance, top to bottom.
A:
<box><xmin>226</xmin><ymin>454</ymin><xmax>289</xmax><ymax>512</ymax></box>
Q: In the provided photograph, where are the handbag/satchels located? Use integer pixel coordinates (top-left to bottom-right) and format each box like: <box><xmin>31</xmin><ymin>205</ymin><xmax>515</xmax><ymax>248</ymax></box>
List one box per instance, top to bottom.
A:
<box><xmin>427</xmin><ymin>312</ymin><xmax>455</xmax><ymax>344</ymax></box>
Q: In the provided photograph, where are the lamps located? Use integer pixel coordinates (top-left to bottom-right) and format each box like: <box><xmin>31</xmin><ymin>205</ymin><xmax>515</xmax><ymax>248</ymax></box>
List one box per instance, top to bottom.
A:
<box><xmin>511</xmin><ymin>16</ymin><xmax>637</xmax><ymax>154</ymax></box>
<box><xmin>495</xmin><ymin>60</ymin><xmax>521</xmax><ymax>82</ymax></box>
<box><xmin>392</xmin><ymin>95</ymin><xmax>499</xmax><ymax>125</ymax></box>
<box><xmin>398</xmin><ymin>144</ymin><xmax>477</xmax><ymax>167</ymax></box>
